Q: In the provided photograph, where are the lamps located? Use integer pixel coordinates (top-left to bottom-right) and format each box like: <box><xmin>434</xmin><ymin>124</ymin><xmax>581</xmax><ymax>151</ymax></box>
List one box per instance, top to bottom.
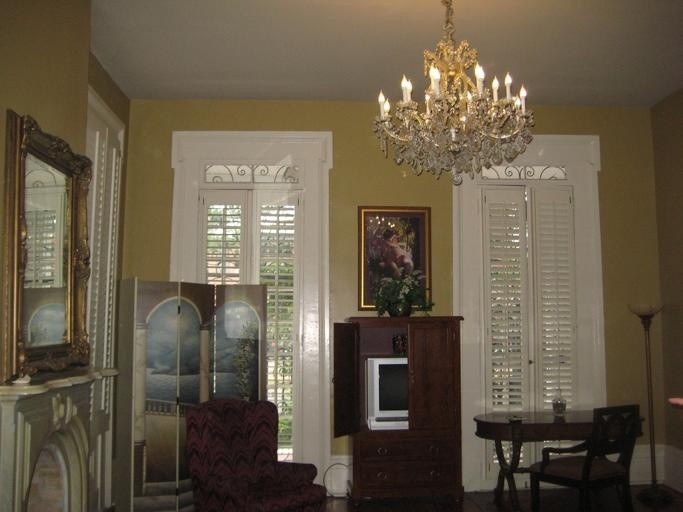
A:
<box><xmin>373</xmin><ymin>2</ymin><xmax>534</xmax><ymax>186</ymax></box>
<box><xmin>628</xmin><ymin>303</ymin><xmax>673</xmax><ymax>507</ymax></box>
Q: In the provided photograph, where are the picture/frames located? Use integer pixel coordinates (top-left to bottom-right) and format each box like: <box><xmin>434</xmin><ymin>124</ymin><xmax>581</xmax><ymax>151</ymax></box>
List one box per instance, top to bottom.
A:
<box><xmin>358</xmin><ymin>205</ymin><xmax>432</xmax><ymax>311</ymax></box>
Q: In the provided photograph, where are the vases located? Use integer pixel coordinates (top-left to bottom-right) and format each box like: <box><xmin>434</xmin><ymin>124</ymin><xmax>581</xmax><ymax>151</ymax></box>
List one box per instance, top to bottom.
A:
<box><xmin>389</xmin><ymin>303</ymin><xmax>411</xmax><ymax>317</ymax></box>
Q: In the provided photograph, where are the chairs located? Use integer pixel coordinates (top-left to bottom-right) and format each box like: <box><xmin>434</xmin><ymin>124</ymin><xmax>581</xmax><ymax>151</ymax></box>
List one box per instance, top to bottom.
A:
<box><xmin>185</xmin><ymin>398</ymin><xmax>326</xmax><ymax>512</ymax></box>
<box><xmin>528</xmin><ymin>405</ymin><xmax>639</xmax><ymax>512</ymax></box>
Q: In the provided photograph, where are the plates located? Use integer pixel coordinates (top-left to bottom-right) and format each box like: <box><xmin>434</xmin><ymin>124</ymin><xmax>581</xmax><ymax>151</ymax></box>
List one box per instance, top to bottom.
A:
<box><xmin>508</xmin><ymin>417</ymin><xmax>523</xmax><ymax>424</ymax></box>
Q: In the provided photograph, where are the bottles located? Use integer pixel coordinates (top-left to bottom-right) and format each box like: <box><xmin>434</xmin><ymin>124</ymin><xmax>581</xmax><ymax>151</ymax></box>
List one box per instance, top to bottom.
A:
<box><xmin>552</xmin><ymin>388</ymin><xmax>567</xmax><ymax>417</ymax></box>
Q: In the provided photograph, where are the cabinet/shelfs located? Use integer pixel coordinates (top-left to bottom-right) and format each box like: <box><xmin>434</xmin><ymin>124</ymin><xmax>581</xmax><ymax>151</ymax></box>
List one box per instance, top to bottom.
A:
<box><xmin>333</xmin><ymin>316</ymin><xmax>464</xmax><ymax>507</ymax></box>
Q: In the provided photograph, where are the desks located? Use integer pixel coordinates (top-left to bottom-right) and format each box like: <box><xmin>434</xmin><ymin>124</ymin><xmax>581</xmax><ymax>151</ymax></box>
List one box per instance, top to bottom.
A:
<box><xmin>473</xmin><ymin>411</ymin><xmax>645</xmax><ymax>512</ymax></box>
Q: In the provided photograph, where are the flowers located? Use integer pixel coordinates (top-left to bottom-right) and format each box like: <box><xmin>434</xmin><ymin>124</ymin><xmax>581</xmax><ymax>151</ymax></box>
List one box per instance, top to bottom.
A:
<box><xmin>373</xmin><ymin>268</ymin><xmax>434</xmax><ymax>316</ymax></box>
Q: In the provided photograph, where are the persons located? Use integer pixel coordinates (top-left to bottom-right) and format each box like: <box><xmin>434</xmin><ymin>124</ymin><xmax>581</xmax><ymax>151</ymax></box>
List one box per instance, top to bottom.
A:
<box><xmin>377</xmin><ymin>228</ymin><xmax>415</xmax><ymax>278</ymax></box>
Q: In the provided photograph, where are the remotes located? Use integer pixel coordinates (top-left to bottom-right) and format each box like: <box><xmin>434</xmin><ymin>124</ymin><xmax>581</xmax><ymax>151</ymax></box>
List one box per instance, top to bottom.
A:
<box><xmin>509</xmin><ymin>416</ymin><xmax>522</xmax><ymax>422</ymax></box>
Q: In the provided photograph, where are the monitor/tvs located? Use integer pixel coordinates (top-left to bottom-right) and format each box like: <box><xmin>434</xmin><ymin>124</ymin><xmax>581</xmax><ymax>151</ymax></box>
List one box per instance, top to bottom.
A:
<box><xmin>364</xmin><ymin>357</ymin><xmax>409</xmax><ymax>430</ymax></box>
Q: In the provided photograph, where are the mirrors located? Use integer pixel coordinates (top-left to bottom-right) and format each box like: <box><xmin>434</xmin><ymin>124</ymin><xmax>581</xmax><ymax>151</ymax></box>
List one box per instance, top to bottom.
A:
<box><xmin>1</xmin><ymin>109</ymin><xmax>90</xmax><ymax>386</ymax></box>
